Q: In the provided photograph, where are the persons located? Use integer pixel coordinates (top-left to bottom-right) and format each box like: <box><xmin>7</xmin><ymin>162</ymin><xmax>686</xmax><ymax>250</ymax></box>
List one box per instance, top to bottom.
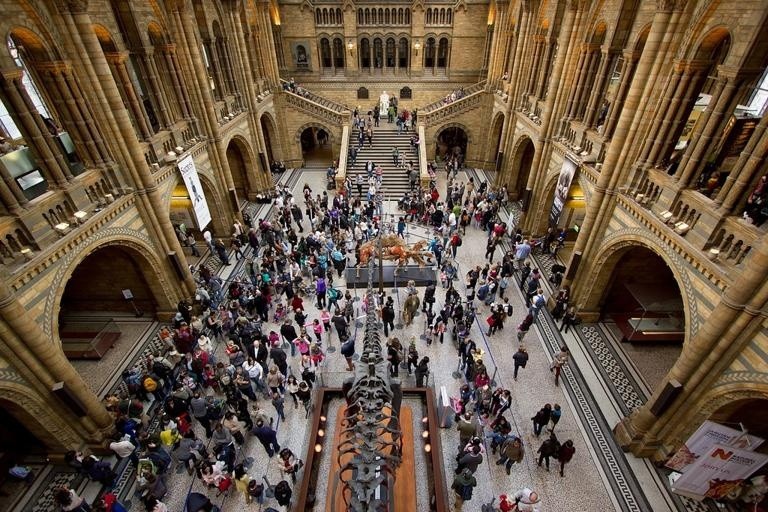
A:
<box><xmin>0</xmin><ymin>74</ymin><xmax>584</xmax><ymax>509</ymax></box>
<box><xmin>597</xmin><ymin>99</ymin><xmax>768</xmax><ymax>255</ymax></box>
<box><xmin>713</xmin><ymin>472</ymin><xmax>768</xmax><ymax>509</ymax></box>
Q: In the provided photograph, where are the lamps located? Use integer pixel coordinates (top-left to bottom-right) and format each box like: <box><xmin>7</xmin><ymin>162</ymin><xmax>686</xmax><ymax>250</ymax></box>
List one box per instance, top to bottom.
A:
<box><xmin>347</xmin><ymin>39</ymin><xmax>355</xmax><ymax>56</ymax></box>
<box><xmin>414</xmin><ymin>39</ymin><xmax>421</xmax><ymax>56</ymax></box>
